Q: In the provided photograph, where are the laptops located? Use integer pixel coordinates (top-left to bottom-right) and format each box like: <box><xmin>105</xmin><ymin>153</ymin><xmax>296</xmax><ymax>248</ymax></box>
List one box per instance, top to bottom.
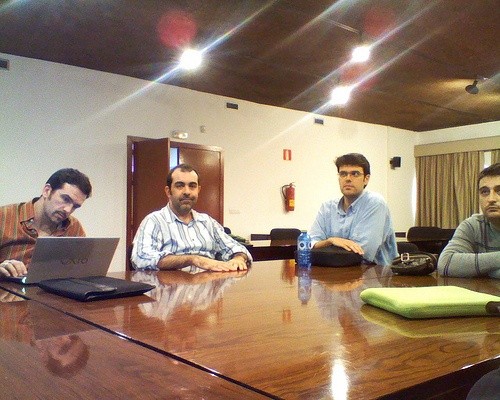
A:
<box><xmin>5</xmin><ymin>237</ymin><xmax>120</xmax><ymax>284</ymax></box>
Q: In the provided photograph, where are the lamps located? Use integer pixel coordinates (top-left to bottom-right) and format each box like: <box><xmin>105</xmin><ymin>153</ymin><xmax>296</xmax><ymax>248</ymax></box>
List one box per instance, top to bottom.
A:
<box><xmin>353</xmin><ymin>27</ymin><xmax>373</xmax><ymax>64</ymax></box>
<box><xmin>465</xmin><ymin>76</ymin><xmax>481</xmax><ymax>94</ymax></box>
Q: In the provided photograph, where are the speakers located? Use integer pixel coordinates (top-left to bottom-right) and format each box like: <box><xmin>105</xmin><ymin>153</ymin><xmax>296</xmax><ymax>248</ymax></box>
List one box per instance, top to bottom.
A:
<box><xmin>390</xmin><ymin>157</ymin><xmax>401</xmax><ymax>167</ymax></box>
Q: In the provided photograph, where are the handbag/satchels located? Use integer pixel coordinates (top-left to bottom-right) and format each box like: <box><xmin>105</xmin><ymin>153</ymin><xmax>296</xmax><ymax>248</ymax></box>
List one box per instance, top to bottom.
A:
<box><xmin>294</xmin><ymin>246</ymin><xmax>364</xmax><ymax>267</ymax></box>
<box><xmin>391</xmin><ymin>251</ymin><xmax>438</xmax><ymax>275</ymax></box>
<box><xmin>38</xmin><ymin>274</ymin><xmax>156</xmax><ymax>301</ymax></box>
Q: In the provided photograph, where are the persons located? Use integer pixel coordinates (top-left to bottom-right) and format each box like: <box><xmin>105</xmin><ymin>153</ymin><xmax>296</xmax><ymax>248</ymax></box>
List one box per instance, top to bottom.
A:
<box><xmin>305</xmin><ymin>152</ymin><xmax>401</xmax><ymax>268</ymax></box>
<box><xmin>436</xmin><ymin>161</ymin><xmax>500</xmax><ymax>281</ymax></box>
<box><xmin>130</xmin><ymin>163</ymin><xmax>254</xmax><ymax>275</ymax></box>
<box><xmin>0</xmin><ymin>168</ymin><xmax>93</xmax><ymax>279</ymax></box>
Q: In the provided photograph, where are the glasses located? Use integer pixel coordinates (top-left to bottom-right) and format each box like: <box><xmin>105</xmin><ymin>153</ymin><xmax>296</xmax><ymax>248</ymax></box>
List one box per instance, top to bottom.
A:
<box><xmin>336</xmin><ymin>170</ymin><xmax>367</xmax><ymax>178</ymax></box>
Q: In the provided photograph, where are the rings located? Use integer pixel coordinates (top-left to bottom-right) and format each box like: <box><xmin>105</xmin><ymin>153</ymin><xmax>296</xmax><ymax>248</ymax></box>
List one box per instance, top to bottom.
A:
<box><xmin>3</xmin><ymin>261</ymin><xmax>13</xmax><ymax>269</ymax></box>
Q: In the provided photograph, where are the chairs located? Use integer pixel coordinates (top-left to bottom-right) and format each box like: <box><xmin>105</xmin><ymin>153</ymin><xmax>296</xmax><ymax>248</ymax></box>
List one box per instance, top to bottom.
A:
<box><xmin>406</xmin><ymin>226</ymin><xmax>442</xmax><ymax>237</ymax></box>
<box><xmin>271</xmin><ymin>227</ymin><xmax>300</xmax><ymax>240</ymax></box>
<box><xmin>396</xmin><ymin>231</ymin><xmax>407</xmax><ymax>238</ymax></box>
<box><xmin>396</xmin><ymin>240</ymin><xmax>418</xmax><ymax>255</ymax></box>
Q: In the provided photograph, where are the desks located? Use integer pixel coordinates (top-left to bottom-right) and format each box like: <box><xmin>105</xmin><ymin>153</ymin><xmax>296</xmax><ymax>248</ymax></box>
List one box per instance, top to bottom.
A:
<box><xmin>244</xmin><ymin>236</ymin><xmax>449</xmax><ymax>260</ymax></box>
<box><xmin>0</xmin><ymin>257</ymin><xmax>500</xmax><ymax>400</ymax></box>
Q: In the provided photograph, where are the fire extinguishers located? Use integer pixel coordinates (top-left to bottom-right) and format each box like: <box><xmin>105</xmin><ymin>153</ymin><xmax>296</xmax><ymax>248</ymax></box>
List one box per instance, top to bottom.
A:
<box><xmin>285</xmin><ymin>181</ymin><xmax>295</xmax><ymax>211</ymax></box>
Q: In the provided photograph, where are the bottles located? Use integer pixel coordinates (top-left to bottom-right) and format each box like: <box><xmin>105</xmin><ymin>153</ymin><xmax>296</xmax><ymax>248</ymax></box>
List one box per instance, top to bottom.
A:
<box><xmin>297</xmin><ymin>229</ymin><xmax>312</xmax><ymax>270</ymax></box>
<box><xmin>298</xmin><ymin>270</ymin><xmax>312</xmax><ymax>305</ymax></box>
